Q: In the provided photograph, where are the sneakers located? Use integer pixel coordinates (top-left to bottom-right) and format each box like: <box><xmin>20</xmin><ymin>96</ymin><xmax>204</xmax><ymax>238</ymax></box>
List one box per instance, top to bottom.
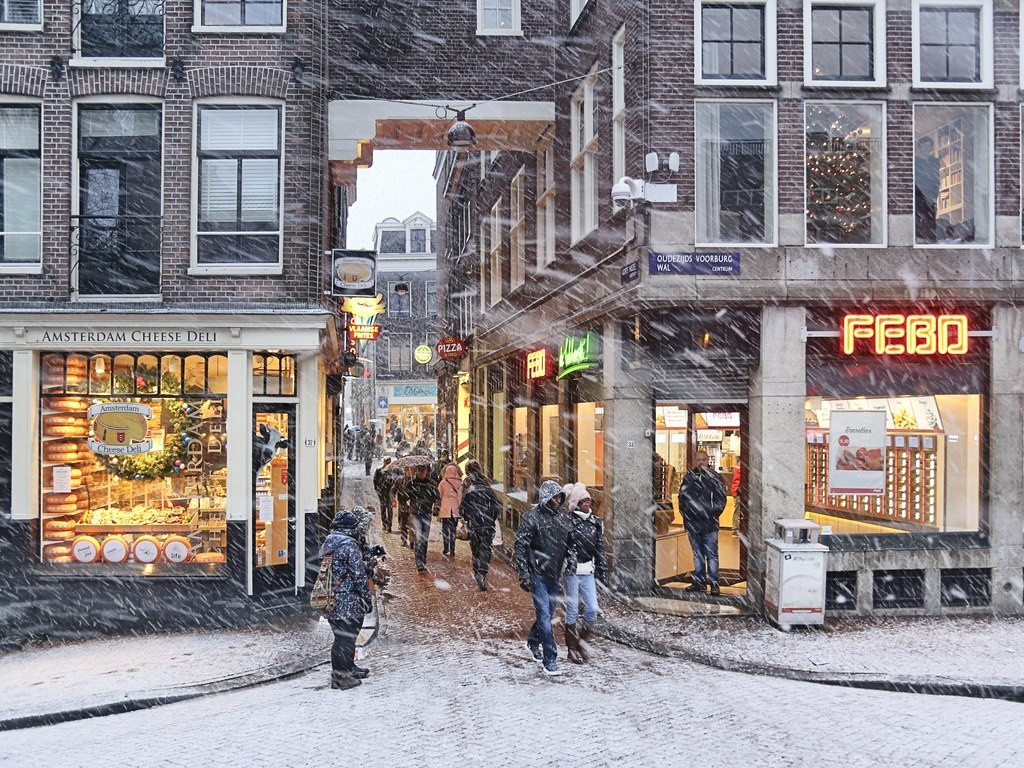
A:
<box><xmin>525</xmin><ymin>640</ymin><xmax>544</xmax><ymax>662</ymax></box>
<box><xmin>544</xmin><ymin>662</ymin><xmax>563</xmax><ymax>675</ymax></box>
<box><xmin>352</xmin><ymin>663</ymin><xmax>370</xmax><ymax>678</ymax></box>
<box><xmin>331</xmin><ymin>670</ymin><xmax>361</xmax><ymax>690</ymax></box>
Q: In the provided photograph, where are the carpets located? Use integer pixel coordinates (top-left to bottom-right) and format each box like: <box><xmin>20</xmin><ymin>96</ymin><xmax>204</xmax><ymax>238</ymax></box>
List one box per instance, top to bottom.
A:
<box><xmin>674</xmin><ymin>568</ymin><xmax>738</xmax><ymax>587</ymax></box>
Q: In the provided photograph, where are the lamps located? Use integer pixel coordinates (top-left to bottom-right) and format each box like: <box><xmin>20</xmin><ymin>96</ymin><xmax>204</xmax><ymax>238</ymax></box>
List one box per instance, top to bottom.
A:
<box><xmin>446</xmin><ymin>102</ymin><xmax>476</xmax><ymax>147</ymax></box>
<box><xmin>395</xmin><ymin>273</ymin><xmax>409</xmax><ymax>295</ymax></box>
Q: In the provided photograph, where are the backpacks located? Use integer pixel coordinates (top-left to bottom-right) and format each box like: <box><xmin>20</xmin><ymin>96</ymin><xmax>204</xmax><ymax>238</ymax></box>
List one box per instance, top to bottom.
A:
<box><xmin>309</xmin><ymin>555</ymin><xmax>334</xmax><ymax>612</ymax></box>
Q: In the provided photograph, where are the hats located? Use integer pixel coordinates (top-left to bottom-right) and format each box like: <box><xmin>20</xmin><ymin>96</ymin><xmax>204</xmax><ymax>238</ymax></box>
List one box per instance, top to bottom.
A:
<box><xmin>441</xmin><ymin>449</ymin><xmax>450</xmax><ymax>455</ymax></box>
<box><xmin>417</xmin><ymin>465</ymin><xmax>427</xmax><ymax>470</ymax></box>
<box><xmin>563</xmin><ymin>482</ymin><xmax>591</xmax><ymax>512</ymax></box>
<box><xmin>330</xmin><ymin>510</ymin><xmax>358</xmax><ymax>530</ymax></box>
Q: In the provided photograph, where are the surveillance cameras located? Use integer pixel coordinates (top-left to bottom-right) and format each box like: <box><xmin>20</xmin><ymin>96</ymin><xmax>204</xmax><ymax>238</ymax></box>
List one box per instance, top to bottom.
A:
<box><xmin>611</xmin><ymin>183</ymin><xmax>631</xmax><ymax>207</ymax></box>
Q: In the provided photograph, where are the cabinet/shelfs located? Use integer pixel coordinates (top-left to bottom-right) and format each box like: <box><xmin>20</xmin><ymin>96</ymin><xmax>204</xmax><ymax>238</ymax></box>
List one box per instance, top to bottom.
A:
<box><xmin>915</xmin><ymin>116</ymin><xmax>973</xmax><ymax>225</ymax></box>
<box><xmin>43</xmin><ymin>355</ymin><xmax>164</xmax><ymax>564</ymax></box>
<box><xmin>805</xmin><ymin>427</ymin><xmax>945</xmax><ymax>528</ymax></box>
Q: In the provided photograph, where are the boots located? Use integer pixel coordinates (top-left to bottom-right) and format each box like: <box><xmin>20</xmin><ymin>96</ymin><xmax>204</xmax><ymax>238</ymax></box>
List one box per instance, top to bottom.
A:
<box><xmin>577</xmin><ymin>617</ymin><xmax>597</xmax><ymax>643</ymax></box>
<box><xmin>564</xmin><ymin>623</ymin><xmax>584</xmax><ymax>664</ymax></box>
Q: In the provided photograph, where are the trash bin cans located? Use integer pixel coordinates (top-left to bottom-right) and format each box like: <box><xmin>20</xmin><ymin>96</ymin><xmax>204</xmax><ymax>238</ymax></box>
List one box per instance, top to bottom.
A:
<box><xmin>764</xmin><ymin>519</ymin><xmax>828</xmax><ymax>632</ymax></box>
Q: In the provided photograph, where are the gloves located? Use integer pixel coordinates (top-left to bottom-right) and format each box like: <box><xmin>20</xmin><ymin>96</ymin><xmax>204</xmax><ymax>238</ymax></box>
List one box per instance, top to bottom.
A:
<box><xmin>433</xmin><ymin>506</ymin><xmax>440</xmax><ymax>516</ymax></box>
<box><xmin>565</xmin><ymin>566</ymin><xmax>576</xmax><ymax>576</ymax></box>
<box><xmin>598</xmin><ymin>571</ymin><xmax>606</xmax><ymax>578</ymax></box>
<box><xmin>520</xmin><ymin>579</ymin><xmax>531</xmax><ymax>592</ymax></box>
<box><xmin>365</xmin><ymin>598</ymin><xmax>372</xmax><ymax>613</ymax></box>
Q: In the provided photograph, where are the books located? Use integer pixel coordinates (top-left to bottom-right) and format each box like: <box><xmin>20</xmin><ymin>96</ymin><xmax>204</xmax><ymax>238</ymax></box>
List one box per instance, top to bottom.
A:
<box><xmin>938</xmin><ymin>128</ymin><xmax>961</xmax><ymax>209</ymax></box>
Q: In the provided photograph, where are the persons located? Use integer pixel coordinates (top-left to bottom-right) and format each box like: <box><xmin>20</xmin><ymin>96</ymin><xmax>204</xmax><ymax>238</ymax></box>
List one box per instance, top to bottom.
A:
<box><xmin>915</xmin><ymin>136</ymin><xmax>940</xmax><ymax>244</ymax></box>
<box><xmin>421</xmin><ymin>416</ymin><xmax>434</xmax><ymax>448</ymax></box>
<box><xmin>343</xmin><ymin>418</ymin><xmax>404</xmax><ymax>461</ymax></box>
<box><xmin>373</xmin><ymin>439</ymin><xmax>489</xmax><ymax>574</ymax></box>
<box><xmin>361</xmin><ymin>440</ymin><xmax>374</xmax><ymax>475</ymax></box>
<box><xmin>678</xmin><ymin>451</ymin><xmax>727</xmax><ymax>596</ymax></box>
<box><xmin>656</xmin><ymin>453</ymin><xmax>675</xmax><ymax>500</ymax></box>
<box><xmin>732</xmin><ymin>456</ymin><xmax>741</xmax><ymax>537</ymax></box>
<box><xmin>459</xmin><ymin>473</ymin><xmax>499</xmax><ymax>591</ymax></box>
<box><xmin>515</xmin><ymin>480</ymin><xmax>608</xmax><ymax>676</ymax></box>
<box><xmin>318</xmin><ymin>506</ymin><xmax>390</xmax><ymax>691</ymax></box>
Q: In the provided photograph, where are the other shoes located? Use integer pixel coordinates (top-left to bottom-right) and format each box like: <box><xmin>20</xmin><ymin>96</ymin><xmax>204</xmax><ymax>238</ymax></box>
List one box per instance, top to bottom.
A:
<box><xmin>382</xmin><ymin>523</ymin><xmax>391</xmax><ymax>532</ymax></box>
<box><xmin>730</xmin><ymin>531</ymin><xmax>741</xmax><ymax>537</ymax></box>
<box><xmin>417</xmin><ymin>562</ymin><xmax>428</xmax><ymax>572</ymax></box>
<box><xmin>685</xmin><ymin>576</ymin><xmax>707</xmax><ymax>591</ymax></box>
<box><xmin>477</xmin><ymin>578</ymin><xmax>487</xmax><ymax>591</ymax></box>
<box><xmin>710</xmin><ymin>581</ymin><xmax>720</xmax><ymax>595</ymax></box>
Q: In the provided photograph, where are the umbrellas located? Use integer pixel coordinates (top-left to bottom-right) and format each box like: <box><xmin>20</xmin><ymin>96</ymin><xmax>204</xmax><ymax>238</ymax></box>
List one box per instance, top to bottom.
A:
<box><xmin>363</xmin><ymin>419</ymin><xmax>384</xmax><ymax>426</ymax></box>
<box><xmin>379</xmin><ymin>455</ymin><xmax>438</xmax><ymax>484</ymax></box>
